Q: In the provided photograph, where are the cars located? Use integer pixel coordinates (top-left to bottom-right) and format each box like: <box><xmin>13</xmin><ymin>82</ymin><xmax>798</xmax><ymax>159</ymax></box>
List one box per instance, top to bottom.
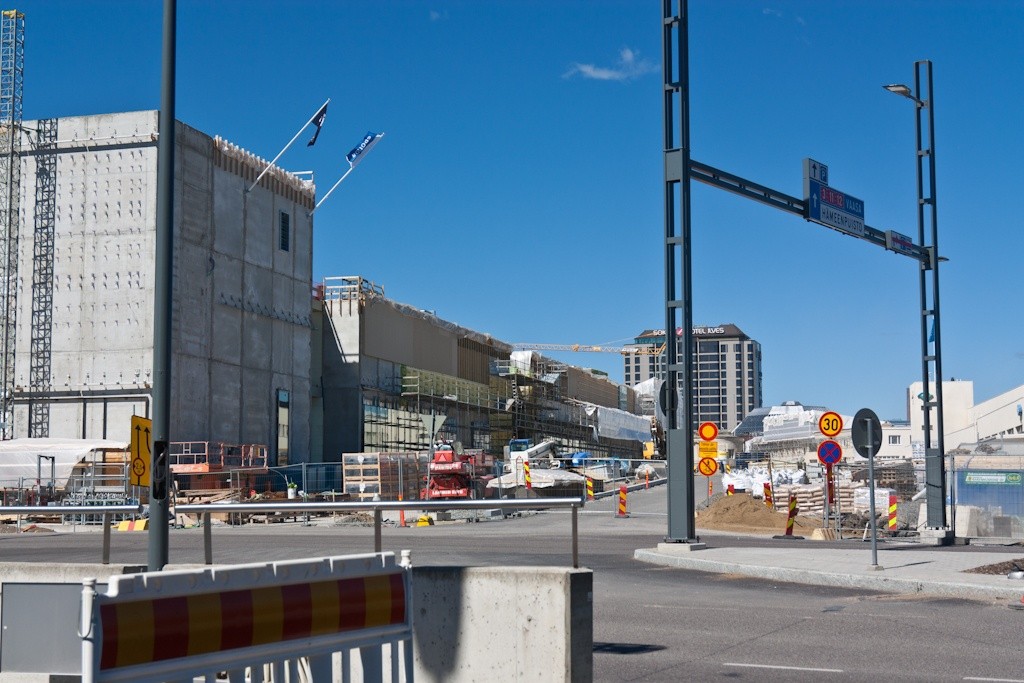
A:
<box><xmin>637</xmin><ymin>464</ymin><xmax>656</xmax><ymax>480</ymax></box>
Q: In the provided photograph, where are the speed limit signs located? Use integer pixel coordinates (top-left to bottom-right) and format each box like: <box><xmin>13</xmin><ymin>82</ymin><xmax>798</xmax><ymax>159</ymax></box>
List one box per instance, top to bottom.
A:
<box><xmin>819</xmin><ymin>412</ymin><xmax>843</xmax><ymax>436</ymax></box>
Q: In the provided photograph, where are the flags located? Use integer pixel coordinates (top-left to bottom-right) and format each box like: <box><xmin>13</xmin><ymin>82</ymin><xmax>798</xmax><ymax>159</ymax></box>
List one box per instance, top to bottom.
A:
<box><xmin>306</xmin><ymin>106</ymin><xmax>328</xmax><ymax>146</ymax></box>
<box><xmin>346</xmin><ymin>133</ymin><xmax>383</xmax><ymax>168</ymax></box>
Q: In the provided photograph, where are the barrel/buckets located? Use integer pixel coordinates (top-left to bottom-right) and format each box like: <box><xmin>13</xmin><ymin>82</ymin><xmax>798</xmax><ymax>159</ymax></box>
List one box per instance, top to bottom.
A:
<box><xmin>727</xmin><ymin>489</ymin><xmax>745</xmax><ymax>496</ymax></box>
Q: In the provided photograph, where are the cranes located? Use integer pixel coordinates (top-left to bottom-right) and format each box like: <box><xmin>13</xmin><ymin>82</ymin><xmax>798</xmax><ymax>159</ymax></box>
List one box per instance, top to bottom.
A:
<box><xmin>511</xmin><ymin>339</ymin><xmax>665</xmax><ymax>357</ymax></box>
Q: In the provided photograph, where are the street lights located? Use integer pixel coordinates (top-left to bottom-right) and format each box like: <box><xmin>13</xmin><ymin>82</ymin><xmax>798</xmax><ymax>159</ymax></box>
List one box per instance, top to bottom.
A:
<box><xmin>883</xmin><ymin>59</ymin><xmax>947</xmax><ymax>529</ymax></box>
